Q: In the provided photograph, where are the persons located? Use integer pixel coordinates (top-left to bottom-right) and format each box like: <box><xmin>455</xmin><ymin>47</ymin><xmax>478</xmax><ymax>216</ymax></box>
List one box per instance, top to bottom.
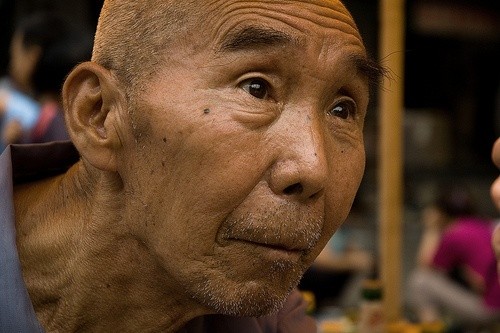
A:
<box><xmin>0</xmin><ymin>1</ymin><xmax>500</xmax><ymax>333</ymax></box>
